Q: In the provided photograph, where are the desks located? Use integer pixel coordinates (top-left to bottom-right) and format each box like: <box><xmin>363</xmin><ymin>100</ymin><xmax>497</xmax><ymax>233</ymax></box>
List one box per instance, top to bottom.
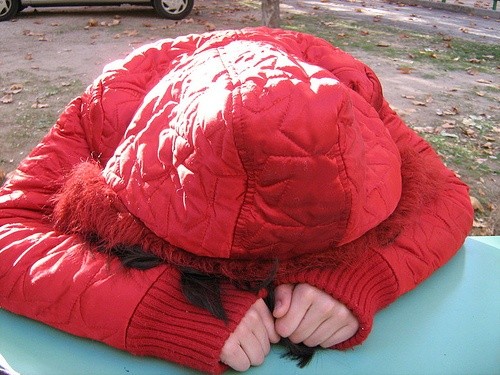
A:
<box><xmin>1</xmin><ymin>233</ymin><xmax>500</xmax><ymax>375</ymax></box>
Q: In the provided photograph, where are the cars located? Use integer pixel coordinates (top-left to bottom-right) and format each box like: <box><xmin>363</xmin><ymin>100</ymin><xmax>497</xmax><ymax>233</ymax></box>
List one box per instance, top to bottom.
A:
<box><xmin>0</xmin><ymin>0</ymin><xmax>197</xmax><ymax>21</ymax></box>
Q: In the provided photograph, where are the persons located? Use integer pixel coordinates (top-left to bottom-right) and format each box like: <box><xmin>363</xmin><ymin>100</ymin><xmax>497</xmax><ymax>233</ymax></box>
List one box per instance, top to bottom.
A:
<box><xmin>0</xmin><ymin>26</ymin><xmax>476</xmax><ymax>374</ymax></box>
